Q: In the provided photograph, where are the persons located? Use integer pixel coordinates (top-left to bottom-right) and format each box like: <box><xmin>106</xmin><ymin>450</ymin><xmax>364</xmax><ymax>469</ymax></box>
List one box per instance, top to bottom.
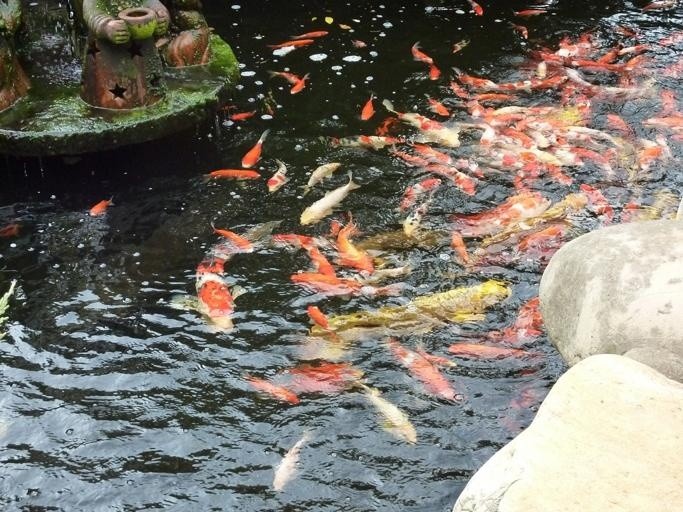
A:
<box><xmin>80</xmin><ymin>0</ymin><xmax>172</xmax><ymax>112</ymax></box>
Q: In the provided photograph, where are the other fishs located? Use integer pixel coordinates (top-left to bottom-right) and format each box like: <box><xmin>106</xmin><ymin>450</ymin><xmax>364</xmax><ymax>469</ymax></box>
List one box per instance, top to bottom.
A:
<box><xmin>165</xmin><ymin>1</ymin><xmax>683</xmax><ymax>404</ymax></box>
